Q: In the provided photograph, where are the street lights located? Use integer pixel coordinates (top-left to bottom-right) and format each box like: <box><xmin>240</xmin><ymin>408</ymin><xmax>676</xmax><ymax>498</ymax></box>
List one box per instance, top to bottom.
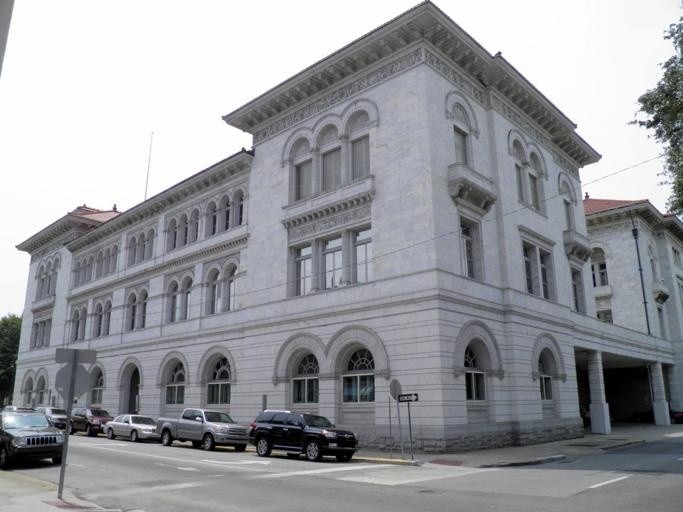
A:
<box><xmin>627</xmin><ymin>208</ymin><xmax>653</xmax><ymax>337</ymax></box>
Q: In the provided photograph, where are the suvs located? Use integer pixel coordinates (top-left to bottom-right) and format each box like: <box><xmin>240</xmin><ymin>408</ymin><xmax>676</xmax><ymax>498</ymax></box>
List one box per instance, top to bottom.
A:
<box><xmin>5</xmin><ymin>406</ymin><xmax>32</xmax><ymax>414</ymax></box>
<box><xmin>38</xmin><ymin>406</ymin><xmax>68</xmax><ymax>428</ymax></box>
<box><xmin>68</xmin><ymin>406</ymin><xmax>111</xmax><ymax>437</ymax></box>
<box><xmin>0</xmin><ymin>410</ymin><xmax>63</xmax><ymax>466</ymax></box>
<box><xmin>247</xmin><ymin>410</ymin><xmax>357</xmax><ymax>465</ymax></box>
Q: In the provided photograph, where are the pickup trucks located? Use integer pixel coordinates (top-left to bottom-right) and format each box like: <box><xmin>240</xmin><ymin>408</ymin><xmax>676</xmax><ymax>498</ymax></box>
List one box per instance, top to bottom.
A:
<box><xmin>155</xmin><ymin>406</ymin><xmax>247</xmax><ymax>452</ymax></box>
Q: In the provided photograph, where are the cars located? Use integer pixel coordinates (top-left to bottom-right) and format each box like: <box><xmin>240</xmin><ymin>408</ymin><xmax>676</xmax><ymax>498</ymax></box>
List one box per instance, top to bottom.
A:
<box><xmin>104</xmin><ymin>413</ymin><xmax>159</xmax><ymax>442</ymax></box>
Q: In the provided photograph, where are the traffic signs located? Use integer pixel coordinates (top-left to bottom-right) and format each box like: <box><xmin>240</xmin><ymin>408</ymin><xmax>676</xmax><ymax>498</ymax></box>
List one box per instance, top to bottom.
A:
<box><xmin>398</xmin><ymin>393</ymin><xmax>419</xmax><ymax>402</ymax></box>
